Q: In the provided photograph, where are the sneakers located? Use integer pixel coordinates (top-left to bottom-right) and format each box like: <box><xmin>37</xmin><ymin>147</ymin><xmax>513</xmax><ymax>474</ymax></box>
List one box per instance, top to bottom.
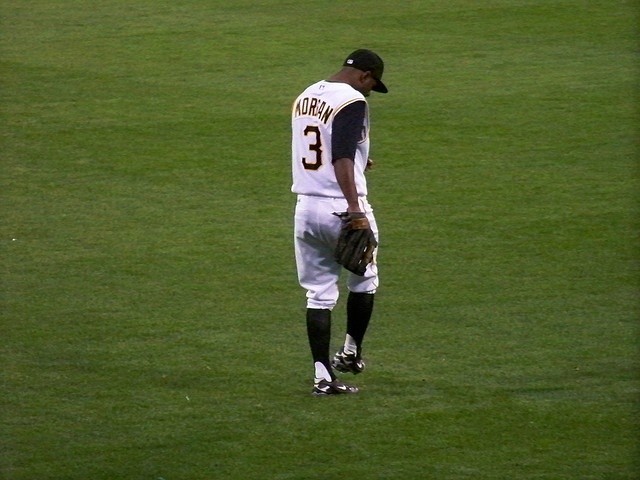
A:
<box><xmin>331</xmin><ymin>347</ymin><xmax>366</xmax><ymax>374</ymax></box>
<box><xmin>311</xmin><ymin>376</ymin><xmax>359</xmax><ymax>397</ymax></box>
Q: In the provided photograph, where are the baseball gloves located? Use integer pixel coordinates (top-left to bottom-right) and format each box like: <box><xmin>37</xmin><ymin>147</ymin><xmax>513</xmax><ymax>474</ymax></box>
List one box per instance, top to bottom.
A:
<box><xmin>333</xmin><ymin>209</ymin><xmax>378</xmax><ymax>278</ymax></box>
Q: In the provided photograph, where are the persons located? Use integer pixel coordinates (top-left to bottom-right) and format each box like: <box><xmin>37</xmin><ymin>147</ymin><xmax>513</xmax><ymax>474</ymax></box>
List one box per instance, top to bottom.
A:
<box><xmin>291</xmin><ymin>48</ymin><xmax>388</xmax><ymax>398</ymax></box>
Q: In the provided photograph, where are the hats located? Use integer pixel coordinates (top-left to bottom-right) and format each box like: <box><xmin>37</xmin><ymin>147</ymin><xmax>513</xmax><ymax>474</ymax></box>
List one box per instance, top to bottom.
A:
<box><xmin>342</xmin><ymin>48</ymin><xmax>388</xmax><ymax>94</ymax></box>
